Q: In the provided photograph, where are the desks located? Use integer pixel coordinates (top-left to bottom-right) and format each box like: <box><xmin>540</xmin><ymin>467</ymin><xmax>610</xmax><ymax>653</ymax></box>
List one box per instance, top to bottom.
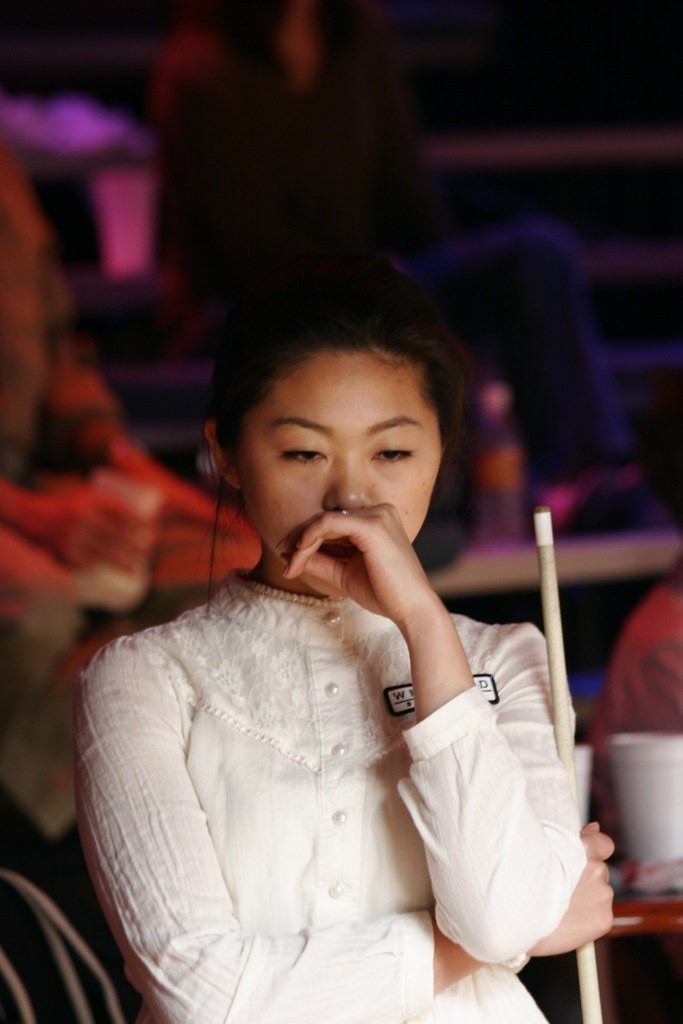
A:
<box><xmin>594</xmin><ymin>896</ymin><xmax>683</xmax><ymax>1024</ymax></box>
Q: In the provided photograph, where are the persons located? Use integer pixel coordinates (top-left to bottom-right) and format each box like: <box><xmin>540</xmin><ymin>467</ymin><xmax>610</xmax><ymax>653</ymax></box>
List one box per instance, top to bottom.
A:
<box><xmin>0</xmin><ymin>0</ymin><xmax>447</xmax><ymax>571</ymax></box>
<box><xmin>76</xmin><ymin>250</ymin><xmax>614</xmax><ymax>1024</ymax></box>
<box><xmin>583</xmin><ymin>373</ymin><xmax>683</xmax><ymax>977</ymax></box>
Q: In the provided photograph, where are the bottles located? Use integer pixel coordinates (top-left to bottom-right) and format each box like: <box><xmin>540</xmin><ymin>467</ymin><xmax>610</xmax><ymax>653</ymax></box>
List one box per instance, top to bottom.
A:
<box><xmin>468</xmin><ymin>384</ymin><xmax>526</xmax><ymax>553</ymax></box>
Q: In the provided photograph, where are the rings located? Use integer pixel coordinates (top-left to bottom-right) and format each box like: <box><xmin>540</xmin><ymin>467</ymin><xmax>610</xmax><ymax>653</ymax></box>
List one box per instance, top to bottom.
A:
<box><xmin>342</xmin><ymin>509</ymin><xmax>349</xmax><ymax>516</ymax></box>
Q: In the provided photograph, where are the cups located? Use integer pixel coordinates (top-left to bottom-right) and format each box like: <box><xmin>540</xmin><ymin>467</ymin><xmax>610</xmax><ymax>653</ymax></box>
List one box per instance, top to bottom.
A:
<box><xmin>92</xmin><ymin>168</ymin><xmax>157</xmax><ymax>281</ymax></box>
<box><xmin>609</xmin><ymin>733</ymin><xmax>683</xmax><ymax>892</ymax></box>
<box><xmin>574</xmin><ymin>742</ymin><xmax>593</xmax><ymax>827</ymax></box>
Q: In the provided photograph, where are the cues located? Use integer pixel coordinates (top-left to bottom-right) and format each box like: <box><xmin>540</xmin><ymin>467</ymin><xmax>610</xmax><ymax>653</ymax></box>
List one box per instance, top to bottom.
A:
<box><xmin>530</xmin><ymin>499</ymin><xmax>605</xmax><ymax>1024</ymax></box>
